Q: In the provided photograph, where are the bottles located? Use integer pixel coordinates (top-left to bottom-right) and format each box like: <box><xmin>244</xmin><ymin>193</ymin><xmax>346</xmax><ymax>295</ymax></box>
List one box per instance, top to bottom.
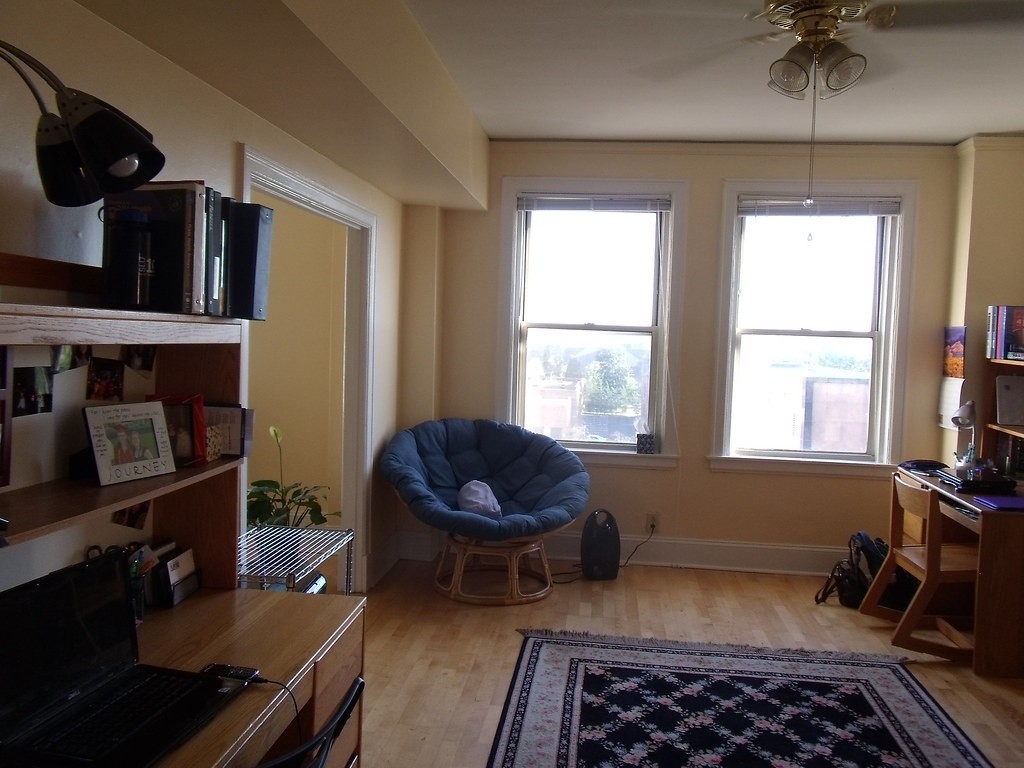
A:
<box><xmin>96</xmin><ymin>203</ymin><xmax>153</xmax><ymax>311</ymax></box>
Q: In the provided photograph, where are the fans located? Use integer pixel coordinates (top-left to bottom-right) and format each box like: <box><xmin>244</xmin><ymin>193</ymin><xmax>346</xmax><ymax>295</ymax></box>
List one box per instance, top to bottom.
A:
<box><xmin>631</xmin><ymin>0</ymin><xmax>1024</xmax><ymax>93</ymax></box>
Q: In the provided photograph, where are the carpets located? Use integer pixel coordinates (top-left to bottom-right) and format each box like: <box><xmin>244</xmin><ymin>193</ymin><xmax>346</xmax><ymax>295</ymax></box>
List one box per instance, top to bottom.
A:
<box><xmin>486</xmin><ymin>629</ymin><xmax>995</xmax><ymax>768</ymax></box>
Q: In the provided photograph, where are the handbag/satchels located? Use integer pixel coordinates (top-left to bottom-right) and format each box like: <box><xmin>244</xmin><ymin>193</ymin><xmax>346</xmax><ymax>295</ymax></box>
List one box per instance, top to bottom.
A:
<box><xmin>815</xmin><ymin>559</ymin><xmax>868</xmax><ymax>608</ymax></box>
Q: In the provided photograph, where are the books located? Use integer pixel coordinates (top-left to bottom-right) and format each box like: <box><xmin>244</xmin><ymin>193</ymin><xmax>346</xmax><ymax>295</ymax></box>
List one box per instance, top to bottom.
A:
<box><xmin>102</xmin><ymin>180</ymin><xmax>275</xmax><ymax>321</ymax></box>
<box><xmin>986</xmin><ymin>305</ymin><xmax>1024</xmax><ymax>362</ymax></box>
<box><xmin>995</xmin><ymin>433</ymin><xmax>1024</xmax><ymax>481</ymax></box>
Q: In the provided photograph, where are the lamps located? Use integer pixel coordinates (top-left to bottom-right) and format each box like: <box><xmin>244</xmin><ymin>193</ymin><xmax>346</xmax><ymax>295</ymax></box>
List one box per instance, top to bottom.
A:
<box><xmin>766</xmin><ymin>17</ymin><xmax>868</xmax><ymax>240</ymax></box>
<box><xmin>0</xmin><ymin>38</ymin><xmax>166</xmax><ymax>206</ymax></box>
<box><xmin>951</xmin><ymin>399</ymin><xmax>978</xmax><ymax>470</ymax></box>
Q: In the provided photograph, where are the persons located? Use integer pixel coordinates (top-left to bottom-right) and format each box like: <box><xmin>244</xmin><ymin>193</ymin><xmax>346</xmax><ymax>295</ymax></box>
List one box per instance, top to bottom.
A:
<box><xmin>113</xmin><ymin>424</ymin><xmax>153</xmax><ymax>463</ymax></box>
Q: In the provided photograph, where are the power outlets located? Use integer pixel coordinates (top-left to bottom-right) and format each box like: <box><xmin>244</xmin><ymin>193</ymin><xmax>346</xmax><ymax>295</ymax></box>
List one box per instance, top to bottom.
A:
<box><xmin>645</xmin><ymin>513</ymin><xmax>660</xmax><ymax>533</ymax></box>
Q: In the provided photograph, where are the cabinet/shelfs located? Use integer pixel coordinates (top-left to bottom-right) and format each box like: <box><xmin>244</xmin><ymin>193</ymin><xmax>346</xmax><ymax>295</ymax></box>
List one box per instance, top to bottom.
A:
<box><xmin>1</xmin><ymin>252</ymin><xmax>248</xmax><ymax>590</ymax></box>
<box><xmin>978</xmin><ymin>357</ymin><xmax>1024</xmax><ymax>468</ymax></box>
<box><xmin>235</xmin><ymin>522</ymin><xmax>353</xmax><ymax>594</ymax></box>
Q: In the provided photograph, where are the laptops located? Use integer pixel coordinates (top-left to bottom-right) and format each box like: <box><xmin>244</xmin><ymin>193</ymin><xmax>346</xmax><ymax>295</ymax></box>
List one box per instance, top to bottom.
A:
<box><xmin>936</xmin><ymin>466</ymin><xmax>1017</xmax><ymax>488</ymax></box>
<box><xmin>0</xmin><ymin>546</ymin><xmax>248</xmax><ymax>768</ymax></box>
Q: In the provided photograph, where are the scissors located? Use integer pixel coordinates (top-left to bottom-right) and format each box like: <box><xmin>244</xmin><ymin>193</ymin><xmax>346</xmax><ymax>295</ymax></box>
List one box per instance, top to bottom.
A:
<box><xmin>87</xmin><ymin>544</ymin><xmax>120</xmax><ymax>559</ymax></box>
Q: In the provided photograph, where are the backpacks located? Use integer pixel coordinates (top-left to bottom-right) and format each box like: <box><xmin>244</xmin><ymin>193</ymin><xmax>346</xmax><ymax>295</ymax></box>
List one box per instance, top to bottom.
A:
<box><xmin>847</xmin><ymin>530</ymin><xmax>898</xmax><ymax>607</ymax></box>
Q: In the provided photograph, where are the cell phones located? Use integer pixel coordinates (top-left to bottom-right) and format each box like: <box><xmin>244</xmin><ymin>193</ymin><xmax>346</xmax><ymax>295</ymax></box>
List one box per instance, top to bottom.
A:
<box><xmin>201</xmin><ymin>662</ymin><xmax>260</xmax><ymax>684</ymax></box>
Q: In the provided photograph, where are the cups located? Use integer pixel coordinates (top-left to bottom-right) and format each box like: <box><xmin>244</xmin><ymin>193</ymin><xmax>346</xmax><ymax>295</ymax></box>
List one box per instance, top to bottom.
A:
<box><xmin>956</xmin><ymin>462</ymin><xmax>973</xmax><ymax>479</ymax></box>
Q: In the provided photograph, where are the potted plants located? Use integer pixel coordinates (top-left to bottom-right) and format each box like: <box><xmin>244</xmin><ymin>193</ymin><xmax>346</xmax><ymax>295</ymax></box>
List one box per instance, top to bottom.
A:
<box><xmin>248</xmin><ymin>426</ymin><xmax>343</xmax><ymax>570</ymax></box>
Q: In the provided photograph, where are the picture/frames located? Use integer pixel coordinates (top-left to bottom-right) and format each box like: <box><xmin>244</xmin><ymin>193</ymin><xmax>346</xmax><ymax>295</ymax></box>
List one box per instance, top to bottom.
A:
<box><xmin>85</xmin><ymin>401</ymin><xmax>176</xmax><ymax>486</ymax></box>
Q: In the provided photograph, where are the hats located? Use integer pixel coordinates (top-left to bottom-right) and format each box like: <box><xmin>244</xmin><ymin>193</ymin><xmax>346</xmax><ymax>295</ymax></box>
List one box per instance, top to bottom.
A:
<box><xmin>113</xmin><ymin>425</ymin><xmax>128</xmax><ymax>434</ymax></box>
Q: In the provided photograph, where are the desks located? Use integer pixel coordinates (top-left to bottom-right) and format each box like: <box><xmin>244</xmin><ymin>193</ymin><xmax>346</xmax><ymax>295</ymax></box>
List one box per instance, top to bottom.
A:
<box><xmin>896</xmin><ymin>466</ymin><xmax>1023</xmax><ymax>675</ymax></box>
<box><xmin>129</xmin><ymin>587</ymin><xmax>371</xmax><ymax>768</ymax></box>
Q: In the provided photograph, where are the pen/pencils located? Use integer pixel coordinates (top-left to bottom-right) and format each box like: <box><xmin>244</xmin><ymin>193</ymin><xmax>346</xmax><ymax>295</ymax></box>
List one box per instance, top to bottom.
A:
<box><xmin>139</xmin><ymin>548</ymin><xmax>144</xmax><ymax>561</ymax></box>
<box><xmin>140</xmin><ymin>559</ymin><xmax>155</xmax><ymax>572</ymax></box>
<box><xmin>130</xmin><ymin>560</ymin><xmax>138</xmax><ymax>576</ymax></box>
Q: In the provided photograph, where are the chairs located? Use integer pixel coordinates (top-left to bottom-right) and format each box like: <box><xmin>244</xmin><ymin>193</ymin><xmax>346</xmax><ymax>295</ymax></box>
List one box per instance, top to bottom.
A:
<box><xmin>382</xmin><ymin>418</ymin><xmax>591</xmax><ymax>605</ymax></box>
<box><xmin>859</xmin><ymin>472</ymin><xmax>979</xmax><ymax>653</ymax></box>
<box><xmin>265</xmin><ymin>676</ymin><xmax>365</xmax><ymax>768</ymax></box>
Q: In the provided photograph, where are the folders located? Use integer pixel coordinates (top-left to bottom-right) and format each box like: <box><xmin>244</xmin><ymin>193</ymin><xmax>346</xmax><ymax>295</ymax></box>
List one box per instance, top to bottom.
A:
<box><xmin>102</xmin><ymin>181</ymin><xmax>221</xmax><ymax>318</ymax></box>
<box><xmin>232</xmin><ymin>201</ymin><xmax>276</xmax><ymax>321</ymax></box>
